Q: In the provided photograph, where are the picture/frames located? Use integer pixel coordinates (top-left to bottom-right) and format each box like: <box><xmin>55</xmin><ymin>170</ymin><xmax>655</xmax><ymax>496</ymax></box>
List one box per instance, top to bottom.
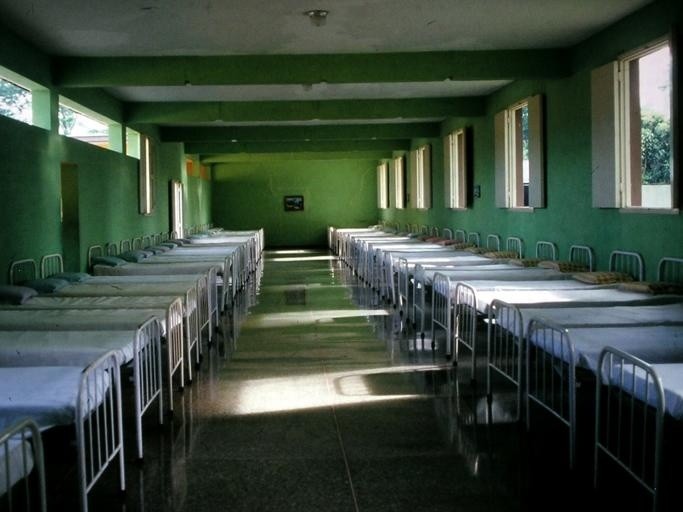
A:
<box><xmin>284</xmin><ymin>195</ymin><xmax>304</xmax><ymax>212</ymax></box>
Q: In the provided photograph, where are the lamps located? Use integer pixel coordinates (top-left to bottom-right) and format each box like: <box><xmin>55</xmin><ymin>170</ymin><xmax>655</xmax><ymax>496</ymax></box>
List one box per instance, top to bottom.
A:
<box><xmin>304</xmin><ymin>7</ymin><xmax>328</xmax><ymax>28</ymax></box>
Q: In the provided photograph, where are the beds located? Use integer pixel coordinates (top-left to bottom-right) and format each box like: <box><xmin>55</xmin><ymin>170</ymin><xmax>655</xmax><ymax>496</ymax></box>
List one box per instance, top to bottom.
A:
<box><xmin>143</xmin><ymin>222</ymin><xmax>264</xmax><ymax>334</ymax></box>
<box><xmin>324</xmin><ymin>222</ymin><xmax>527</xmax><ymax>369</ymax></box>
